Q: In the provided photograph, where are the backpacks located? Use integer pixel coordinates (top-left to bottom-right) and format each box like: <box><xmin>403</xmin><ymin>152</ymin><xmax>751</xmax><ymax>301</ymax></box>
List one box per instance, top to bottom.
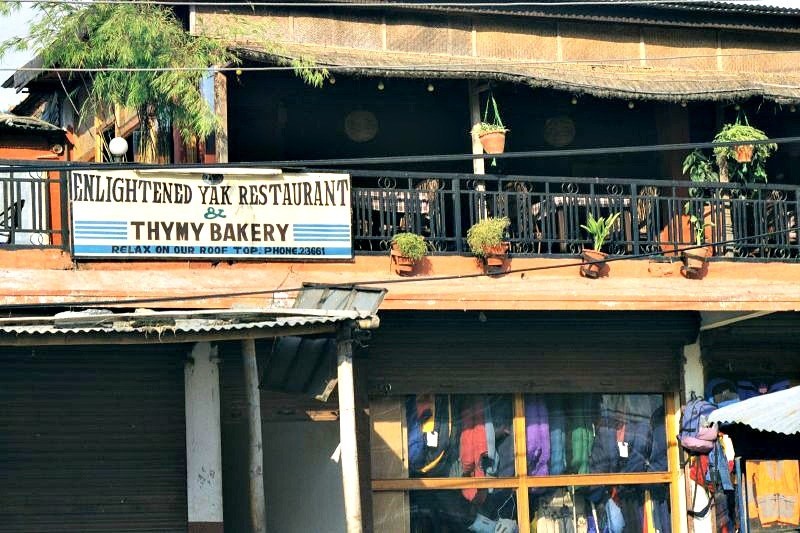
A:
<box><xmin>676</xmin><ymin>392</ymin><xmax>717</xmax><ymax>453</ymax></box>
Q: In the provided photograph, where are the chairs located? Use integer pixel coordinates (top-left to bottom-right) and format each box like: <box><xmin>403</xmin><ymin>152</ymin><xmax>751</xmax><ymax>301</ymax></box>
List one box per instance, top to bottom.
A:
<box><xmin>0</xmin><ymin>199</ymin><xmax>26</xmax><ymax>244</ymax></box>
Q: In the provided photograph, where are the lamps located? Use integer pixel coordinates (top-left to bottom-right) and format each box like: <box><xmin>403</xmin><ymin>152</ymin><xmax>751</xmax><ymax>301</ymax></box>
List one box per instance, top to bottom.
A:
<box><xmin>109</xmin><ymin>137</ymin><xmax>128</xmax><ymax>163</ymax></box>
<box><xmin>52</xmin><ymin>144</ymin><xmax>63</xmax><ymax>155</ymax></box>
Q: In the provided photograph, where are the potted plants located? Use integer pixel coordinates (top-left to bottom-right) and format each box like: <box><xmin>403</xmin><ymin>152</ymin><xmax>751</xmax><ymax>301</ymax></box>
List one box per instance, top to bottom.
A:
<box><xmin>477</xmin><ymin>121</ymin><xmax>507</xmax><ymax>166</ymax></box>
<box><xmin>710</xmin><ymin>123</ymin><xmax>779</xmax><ymax>185</ymax></box>
<box><xmin>579</xmin><ymin>209</ymin><xmax>621</xmax><ymax>278</ymax></box>
<box><xmin>466</xmin><ymin>216</ymin><xmax>512</xmax><ymax>266</ymax></box>
<box><xmin>685</xmin><ymin>216</ymin><xmax>716</xmax><ymax>269</ymax></box>
<box><xmin>388</xmin><ymin>232</ymin><xmax>430</xmax><ymax>274</ymax></box>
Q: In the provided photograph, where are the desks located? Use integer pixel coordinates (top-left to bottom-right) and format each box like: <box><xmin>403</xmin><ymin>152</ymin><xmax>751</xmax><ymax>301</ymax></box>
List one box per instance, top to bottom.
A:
<box><xmin>352</xmin><ymin>188</ymin><xmax>443</xmax><ymax>253</ymax></box>
<box><xmin>531</xmin><ymin>195</ymin><xmax>634</xmax><ymax>254</ymax></box>
